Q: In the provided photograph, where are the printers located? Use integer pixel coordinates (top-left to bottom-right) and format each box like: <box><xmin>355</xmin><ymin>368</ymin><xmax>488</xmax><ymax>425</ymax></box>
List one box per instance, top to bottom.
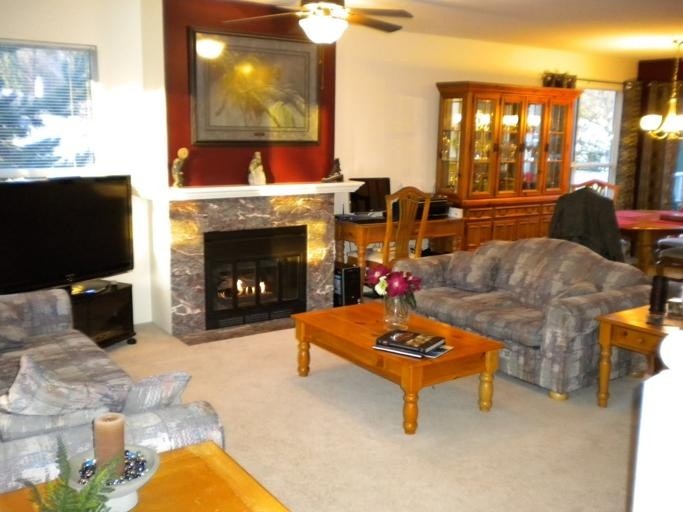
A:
<box><xmin>392</xmin><ymin>198</ymin><xmax>449</xmax><ymax>219</ymax></box>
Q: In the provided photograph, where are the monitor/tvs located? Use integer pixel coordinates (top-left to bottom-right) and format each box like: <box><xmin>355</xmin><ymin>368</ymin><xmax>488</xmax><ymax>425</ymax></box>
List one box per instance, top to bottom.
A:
<box><xmin>0</xmin><ymin>175</ymin><xmax>134</xmax><ymax>296</ymax></box>
<box><xmin>349</xmin><ymin>177</ymin><xmax>390</xmax><ymax>216</ymax></box>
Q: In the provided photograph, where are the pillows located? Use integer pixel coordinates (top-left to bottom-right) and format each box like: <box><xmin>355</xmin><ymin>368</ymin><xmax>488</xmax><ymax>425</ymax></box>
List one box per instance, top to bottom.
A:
<box><xmin>443</xmin><ymin>250</ymin><xmax>501</xmax><ymax>294</ymax></box>
<box><xmin>552</xmin><ymin>278</ymin><xmax>599</xmax><ymax>302</ymax></box>
<box><xmin>0</xmin><ymin>353</ymin><xmax>192</xmax><ymax>418</ymax></box>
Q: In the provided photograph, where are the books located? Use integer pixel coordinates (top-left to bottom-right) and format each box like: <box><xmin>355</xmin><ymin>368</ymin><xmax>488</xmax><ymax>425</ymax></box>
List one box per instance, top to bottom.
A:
<box><xmin>371</xmin><ymin>328</ymin><xmax>455</xmax><ymax>361</ymax></box>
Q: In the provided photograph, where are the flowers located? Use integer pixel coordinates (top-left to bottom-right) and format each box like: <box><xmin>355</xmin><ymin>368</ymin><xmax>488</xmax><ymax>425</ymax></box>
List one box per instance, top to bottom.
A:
<box><xmin>362</xmin><ymin>262</ymin><xmax>421</xmax><ymax>310</ymax></box>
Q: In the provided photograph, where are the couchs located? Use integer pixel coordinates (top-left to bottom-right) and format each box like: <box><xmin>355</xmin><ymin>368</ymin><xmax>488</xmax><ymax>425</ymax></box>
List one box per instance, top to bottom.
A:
<box><xmin>390</xmin><ymin>237</ymin><xmax>653</xmax><ymax>402</ymax></box>
<box><xmin>0</xmin><ymin>287</ymin><xmax>227</xmax><ymax>497</ymax></box>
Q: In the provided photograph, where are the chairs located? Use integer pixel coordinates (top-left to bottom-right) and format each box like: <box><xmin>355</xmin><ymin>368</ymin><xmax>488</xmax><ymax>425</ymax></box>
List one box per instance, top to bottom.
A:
<box><xmin>346</xmin><ymin>176</ymin><xmax>395</xmax><ymax>259</ymax></box>
<box><xmin>573</xmin><ymin>179</ymin><xmax>622</xmax><ymax>209</ymax></box>
<box><xmin>348</xmin><ymin>185</ymin><xmax>437</xmax><ymax>287</ymax></box>
<box><xmin>548</xmin><ymin>187</ymin><xmax>641</xmax><ymax>269</ymax></box>
<box><xmin>653</xmin><ymin>226</ymin><xmax>681</xmax><ymax>289</ymax></box>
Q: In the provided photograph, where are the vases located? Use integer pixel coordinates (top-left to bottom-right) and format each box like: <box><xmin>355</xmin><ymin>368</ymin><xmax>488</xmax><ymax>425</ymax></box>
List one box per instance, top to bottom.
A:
<box><xmin>384</xmin><ymin>294</ymin><xmax>409</xmax><ymax>331</ymax></box>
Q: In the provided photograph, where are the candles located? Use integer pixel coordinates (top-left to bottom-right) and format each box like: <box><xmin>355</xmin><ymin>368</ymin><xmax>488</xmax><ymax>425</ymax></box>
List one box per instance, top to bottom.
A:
<box><xmin>91</xmin><ymin>412</ymin><xmax>126</xmax><ymax>479</ymax></box>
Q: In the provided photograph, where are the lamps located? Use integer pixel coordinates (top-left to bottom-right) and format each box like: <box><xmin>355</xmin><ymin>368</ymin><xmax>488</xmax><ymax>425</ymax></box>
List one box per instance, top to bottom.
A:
<box><xmin>296</xmin><ymin>0</ymin><xmax>352</xmax><ymax>45</ymax></box>
<box><xmin>639</xmin><ymin>35</ymin><xmax>682</xmax><ymax>146</ymax></box>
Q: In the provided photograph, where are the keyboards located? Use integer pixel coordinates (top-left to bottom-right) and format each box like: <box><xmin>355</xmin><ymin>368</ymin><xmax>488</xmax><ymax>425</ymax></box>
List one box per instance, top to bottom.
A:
<box><xmin>353</xmin><ymin>216</ymin><xmax>396</xmax><ymax>223</ymax></box>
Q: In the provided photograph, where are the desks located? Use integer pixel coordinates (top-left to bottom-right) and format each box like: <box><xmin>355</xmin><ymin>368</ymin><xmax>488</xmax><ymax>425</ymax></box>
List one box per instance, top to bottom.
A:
<box><xmin>613</xmin><ymin>210</ymin><xmax>682</xmax><ymax>275</ymax></box>
<box><xmin>333</xmin><ymin>213</ymin><xmax>466</xmax><ymax>303</ymax></box>
<box><xmin>289</xmin><ymin>299</ymin><xmax>505</xmax><ymax>435</ymax></box>
<box><xmin>594</xmin><ymin>302</ymin><xmax>682</xmax><ymax>408</ymax></box>
<box><xmin>0</xmin><ymin>438</ymin><xmax>293</xmax><ymax>512</ymax></box>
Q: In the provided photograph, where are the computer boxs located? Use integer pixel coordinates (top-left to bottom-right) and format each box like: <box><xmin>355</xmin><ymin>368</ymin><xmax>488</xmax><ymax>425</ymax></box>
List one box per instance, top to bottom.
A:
<box><xmin>333</xmin><ymin>261</ymin><xmax>360</xmax><ymax>307</ymax></box>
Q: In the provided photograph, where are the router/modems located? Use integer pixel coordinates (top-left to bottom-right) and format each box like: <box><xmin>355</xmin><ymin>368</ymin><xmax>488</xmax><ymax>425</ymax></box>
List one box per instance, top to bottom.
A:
<box><xmin>335</xmin><ymin>204</ymin><xmax>353</xmax><ymax>220</ymax></box>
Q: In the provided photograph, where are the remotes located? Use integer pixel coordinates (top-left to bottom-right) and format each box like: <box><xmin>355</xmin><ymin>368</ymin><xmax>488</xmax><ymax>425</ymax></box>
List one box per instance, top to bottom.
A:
<box><xmin>111</xmin><ymin>280</ymin><xmax>117</xmax><ymax>286</ymax></box>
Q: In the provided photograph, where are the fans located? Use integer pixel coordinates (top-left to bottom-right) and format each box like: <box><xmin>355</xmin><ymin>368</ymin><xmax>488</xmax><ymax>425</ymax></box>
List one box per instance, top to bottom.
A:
<box><xmin>219</xmin><ymin>0</ymin><xmax>415</xmax><ymax>33</ymax></box>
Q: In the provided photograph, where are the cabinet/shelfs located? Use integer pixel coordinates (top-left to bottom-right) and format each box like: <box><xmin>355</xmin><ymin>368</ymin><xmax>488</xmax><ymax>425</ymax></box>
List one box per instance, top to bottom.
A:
<box><xmin>433</xmin><ymin>80</ymin><xmax>584</xmax><ymax>206</ymax></box>
<box><xmin>434</xmin><ymin>194</ymin><xmax>494</xmax><ymax>259</ymax></box>
<box><xmin>514</xmin><ymin>195</ymin><xmax>542</xmax><ymax>236</ymax></box>
<box><xmin>535</xmin><ymin>196</ymin><xmax>562</xmax><ymax>237</ymax></box>
<box><xmin>490</xmin><ymin>197</ymin><xmax>519</xmax><ymax>240</ymax></box>
<box><xmin>69</xmin><ymin>280</ymin><xmax>137</xmax><ymax>349</ymax></box>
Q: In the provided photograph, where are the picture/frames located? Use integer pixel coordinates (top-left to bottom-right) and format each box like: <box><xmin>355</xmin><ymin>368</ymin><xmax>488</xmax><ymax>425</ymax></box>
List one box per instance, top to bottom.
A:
<box><xmin>186</xmin><ymin>25</ymin><xmax>325</xmax><ymax>147</ymax></box>
